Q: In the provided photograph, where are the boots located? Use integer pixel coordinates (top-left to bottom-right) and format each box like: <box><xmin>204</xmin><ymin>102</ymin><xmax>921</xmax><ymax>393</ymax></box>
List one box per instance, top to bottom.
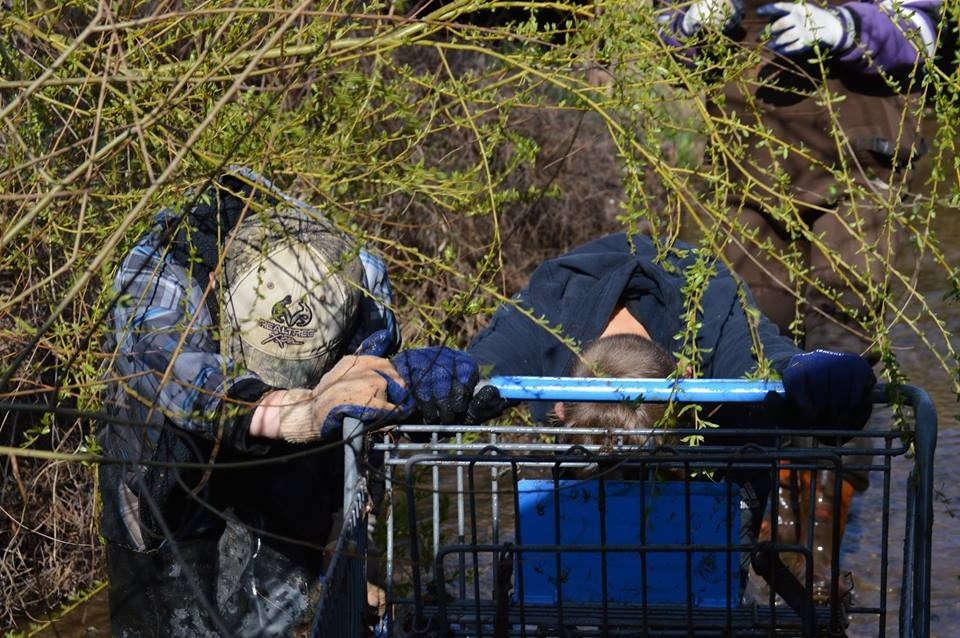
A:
<box><xmin>740</xmin><ymin>460</ymin><xmax>856</xmax><ymax>606</ymax></box>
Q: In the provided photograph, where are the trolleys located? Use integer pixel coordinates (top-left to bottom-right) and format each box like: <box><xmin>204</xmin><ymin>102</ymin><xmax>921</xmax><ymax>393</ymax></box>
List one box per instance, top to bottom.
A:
<box><xmin>309</xmin><ymin>376</ymin><xmax>939</xmax><ymax>638</ymax></box>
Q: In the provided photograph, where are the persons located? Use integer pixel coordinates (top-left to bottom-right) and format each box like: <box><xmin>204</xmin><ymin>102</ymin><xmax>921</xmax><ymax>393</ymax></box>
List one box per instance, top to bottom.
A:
<box><xmin>653</xmin><ymin>1</ymin><xmax>952</xmax><ymax>96</ymax></box>
<box><xmin>100</xmin><ymin>170</ymin><xmax>415</xmax><ymax>638</ymax></box>
<box><xmin>391</xmin><ymin>235</ymin><xmax>876</xmax><ymax>602</ymax></box>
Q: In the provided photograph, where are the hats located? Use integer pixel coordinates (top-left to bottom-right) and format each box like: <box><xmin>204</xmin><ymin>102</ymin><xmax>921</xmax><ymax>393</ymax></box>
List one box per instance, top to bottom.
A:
<box><xmin>218</xmin><ymin>202</ymin><xmax>364</xmax><ymax>392</ymax></box>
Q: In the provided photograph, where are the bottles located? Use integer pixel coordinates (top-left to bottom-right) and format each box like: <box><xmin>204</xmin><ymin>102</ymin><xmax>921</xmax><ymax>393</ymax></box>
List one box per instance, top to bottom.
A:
<box><xmin>748</xmin><ymin>447</ymin><xmax>863</xmax><ymax>608</ymax></box>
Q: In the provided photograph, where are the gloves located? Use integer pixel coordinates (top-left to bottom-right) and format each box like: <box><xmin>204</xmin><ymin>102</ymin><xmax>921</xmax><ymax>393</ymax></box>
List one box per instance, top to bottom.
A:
<box><xmin>680</xmin><ymin>0</ymin><xmax>746</xmax><ymax>39</ymax></box>
<box><xmin>756</xmin><ymin>1</ymin><xmax>843</xmax><ymax>55</ymax></box>
<box><xmin>388</xmin><ymin>347</ymin><xmax>502</xmax><ymax>445</ymax></box>
<box><xmin>765</xmin><ymin>349</ymin><xmax>878</xmax><ymax>447</ymax></box>
<box><xmin>279</xmin><ymin>353</ymin><xmax>406</xmax><ymax>444</ymax></box>
